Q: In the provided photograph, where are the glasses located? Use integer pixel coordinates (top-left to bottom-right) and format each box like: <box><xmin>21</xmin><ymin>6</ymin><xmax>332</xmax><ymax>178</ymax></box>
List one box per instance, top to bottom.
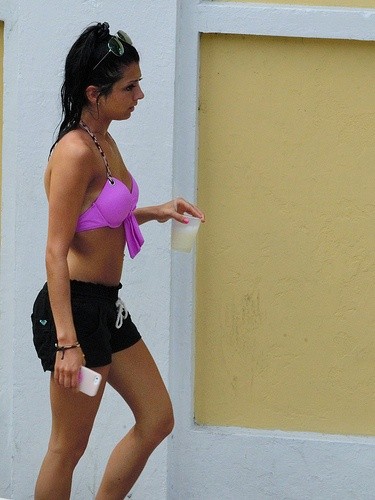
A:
<box><xmin>93</xmin><ymin>30</ymin><xmax>135</xmax><ymax>71</ymax></box>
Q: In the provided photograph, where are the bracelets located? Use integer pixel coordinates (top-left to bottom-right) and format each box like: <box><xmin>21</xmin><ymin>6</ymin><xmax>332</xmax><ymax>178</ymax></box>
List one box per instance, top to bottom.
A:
<box><xmin>54</xmin><ymin>343</ymin><xmax>80</xmax><ymax>360</ymax></box>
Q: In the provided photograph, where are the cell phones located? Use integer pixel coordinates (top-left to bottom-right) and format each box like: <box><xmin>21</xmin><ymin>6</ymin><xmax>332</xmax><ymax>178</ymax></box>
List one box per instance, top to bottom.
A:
<box><xmin>78</xmin><ymin>365</ymin><xmax>102</xmax><ymax>397</ymax></box>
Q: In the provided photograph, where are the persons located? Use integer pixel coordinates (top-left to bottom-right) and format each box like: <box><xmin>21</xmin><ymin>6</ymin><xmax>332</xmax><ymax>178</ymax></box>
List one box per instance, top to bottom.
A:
<box><xmin>32</xmin><ymin>22</ymin><xmax>205</xmax><ymax>500</ymax></box>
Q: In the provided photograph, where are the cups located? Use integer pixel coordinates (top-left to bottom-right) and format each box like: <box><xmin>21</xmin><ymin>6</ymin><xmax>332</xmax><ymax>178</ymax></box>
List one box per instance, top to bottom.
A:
<box><xmin>170</xmin><ymin>215</ymin><xmax>201</xmax><ymax>253</ymax></box>
